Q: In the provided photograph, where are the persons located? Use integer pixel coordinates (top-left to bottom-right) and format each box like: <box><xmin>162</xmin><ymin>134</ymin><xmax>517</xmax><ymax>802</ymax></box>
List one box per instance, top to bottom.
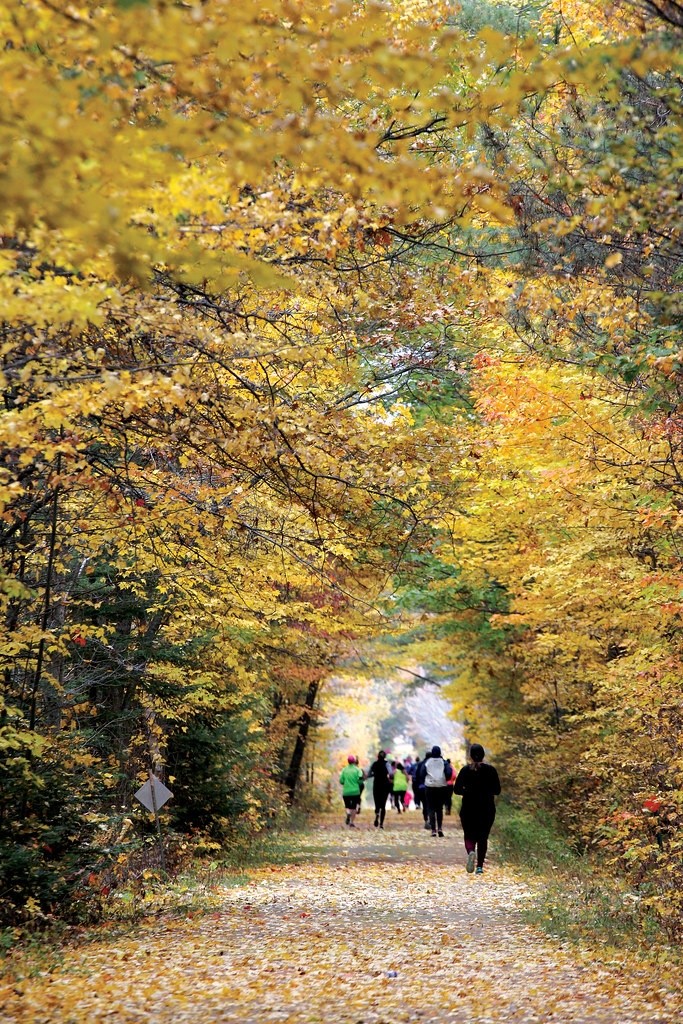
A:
<box><xmin>454</xmin><ymin>743</ymin><xmax>501</xmax><ymax>874</ymax></box>
<box><xmin>340</xmin><ymin>746</ymin><xmax>456</xmax><ymax>837</ymax></box>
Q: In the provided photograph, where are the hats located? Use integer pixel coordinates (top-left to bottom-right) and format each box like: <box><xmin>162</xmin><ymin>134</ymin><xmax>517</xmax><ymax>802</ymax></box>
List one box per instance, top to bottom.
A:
<box><xmin>348</xmin><ymin>755</ymin><xmax>355</xmax><ymax>763</ymax></box>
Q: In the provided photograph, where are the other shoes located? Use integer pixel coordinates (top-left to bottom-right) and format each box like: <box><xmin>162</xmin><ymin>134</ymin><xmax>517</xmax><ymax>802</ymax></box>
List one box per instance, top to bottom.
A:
<box><xmin>345</xmin><ymin>813</ymin><xmax>351</xmax><ymax>824</ymax></box>
<box><xmin>374</xmin><ymin>815</ymin><xmax>379</xmax><ymax>826</ymax></box>
<box><xmin>351</xmin><ymin>824</ymin><xmax>356</xmax><ymax>827</ymax></box>
<box><xmin>438</xmin><ymin>828</ymin><xmax>444</xmax><ymax>837</ymax></box>
<box><xmin>432</xmin><ymin>830</ymin><xmax>436</xmax><ymax>836</ymax></box>
<box><xmin>466</xmin><ymin>851</ymin><xmax>476</xmax><ymax>873</ymax></box>
<box><xmin>476</xmin><ymin>867</ymin><xmax>483</xmax><ymax>874</ymax></box>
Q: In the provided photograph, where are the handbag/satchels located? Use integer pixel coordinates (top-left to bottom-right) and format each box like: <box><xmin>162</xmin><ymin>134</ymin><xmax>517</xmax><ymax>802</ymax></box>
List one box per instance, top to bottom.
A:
<box><xmin>404</xmin><ymin>791</ymin><xmax>412</xmax><ymax>805</ymax></box>
